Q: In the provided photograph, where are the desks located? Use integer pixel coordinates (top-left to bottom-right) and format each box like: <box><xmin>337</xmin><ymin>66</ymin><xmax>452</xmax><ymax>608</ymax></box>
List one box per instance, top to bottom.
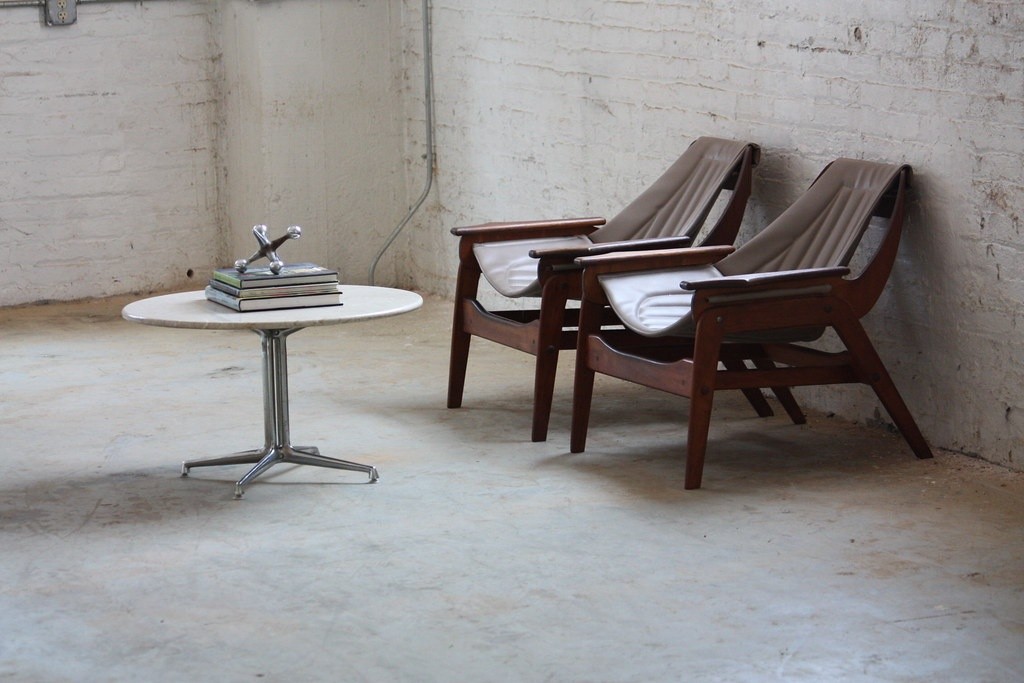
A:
<box><xmin>120</xmin><ymin>284</ymin><xmax>424</xmax><ymax>499</ymax></box>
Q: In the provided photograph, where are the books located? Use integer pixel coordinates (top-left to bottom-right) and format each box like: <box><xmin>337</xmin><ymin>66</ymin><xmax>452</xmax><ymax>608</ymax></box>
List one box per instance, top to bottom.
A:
<box><xmin>205</xmin><ymin>263</ymin><xmax>344</xmax><ymax>312</ymax></box>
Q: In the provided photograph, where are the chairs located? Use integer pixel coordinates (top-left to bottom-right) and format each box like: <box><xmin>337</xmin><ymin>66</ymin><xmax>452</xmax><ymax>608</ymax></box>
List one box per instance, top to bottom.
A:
<box><xmin>447</xmin><ymin>136</ymin><xmax>763</xmax><ymax>443</ymax></box>
<box><xmin>571</xmin><ymin>157</ymin><xmax>934</xmax><ymax>492</ymax></box>
